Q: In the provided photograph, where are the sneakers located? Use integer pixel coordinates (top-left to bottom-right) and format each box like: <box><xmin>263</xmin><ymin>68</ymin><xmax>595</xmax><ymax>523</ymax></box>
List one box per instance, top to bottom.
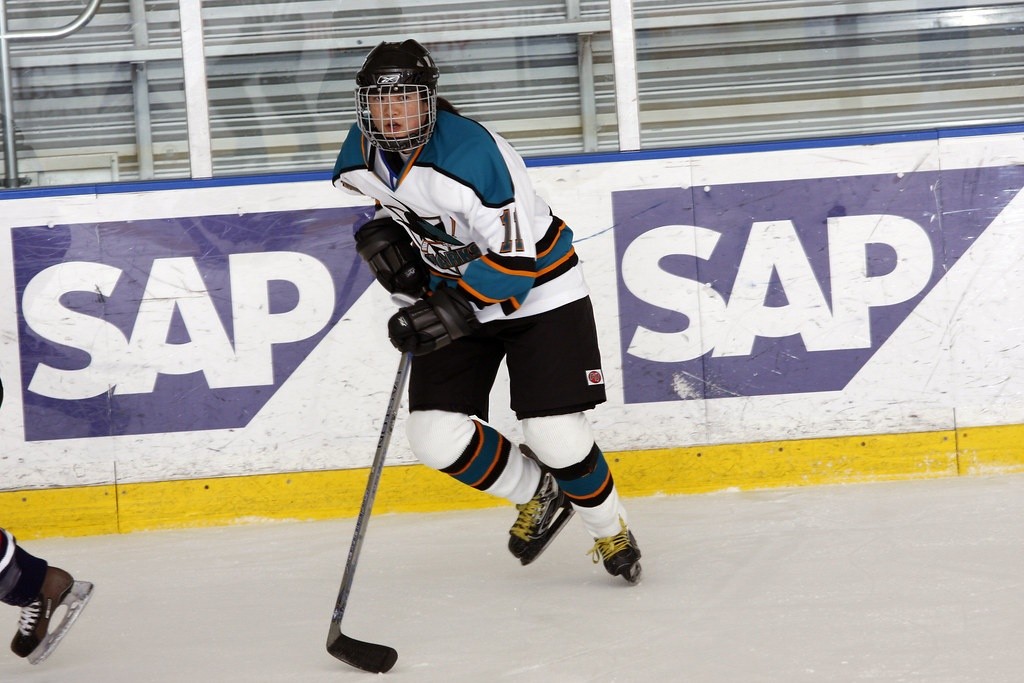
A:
<box><xmin>507</xmin><ymin>444</ymin><xmax>577</xmax><ymax>567</ymax></box>
<box><xmin>585</xmin><ymin>514</ymin><xmax>642</xmax><ymax>585</ymax></box>
<box><xmin>0</xmin><ymin>527</ymin><xmax>96</xmax><ymax>666</ymax></box>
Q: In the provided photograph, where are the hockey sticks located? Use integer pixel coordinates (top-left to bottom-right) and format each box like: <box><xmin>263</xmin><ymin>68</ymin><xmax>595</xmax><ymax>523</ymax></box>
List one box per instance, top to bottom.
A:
<box><xmin>324</xmin><ymin>351</ymin><xmax>412</xmax><ymax>674</ymax></box>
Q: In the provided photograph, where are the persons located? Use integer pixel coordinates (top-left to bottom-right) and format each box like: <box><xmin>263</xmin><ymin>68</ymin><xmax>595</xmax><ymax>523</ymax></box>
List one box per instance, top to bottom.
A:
<box><xmin>331</xmin><ymin>38</ymin><xmax>644</xmax><ymax>583</ymax></box>
<box><xmin>0</xmin><ymin>377</ymin><xmax>94</xmax><ymax>666</ymax></box>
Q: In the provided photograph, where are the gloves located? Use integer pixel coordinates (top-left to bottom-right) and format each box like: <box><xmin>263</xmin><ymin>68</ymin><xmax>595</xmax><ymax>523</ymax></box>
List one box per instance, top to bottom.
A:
<box><xmin>352</xmin><ymin>216</ymin><xmax>432</xmax><ymax>300</ymax></box>
<box><xmin>387</xmin><ymin>286</ymin><xmax>485</xmax><ymax>359</ymax></box>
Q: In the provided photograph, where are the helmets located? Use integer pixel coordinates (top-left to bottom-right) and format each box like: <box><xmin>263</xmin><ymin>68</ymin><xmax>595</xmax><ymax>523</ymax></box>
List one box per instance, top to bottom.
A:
<box><xmin>353</xmin><ymin>38</ymin><xmax>440</xmax><ymax>152</ymax></box>
